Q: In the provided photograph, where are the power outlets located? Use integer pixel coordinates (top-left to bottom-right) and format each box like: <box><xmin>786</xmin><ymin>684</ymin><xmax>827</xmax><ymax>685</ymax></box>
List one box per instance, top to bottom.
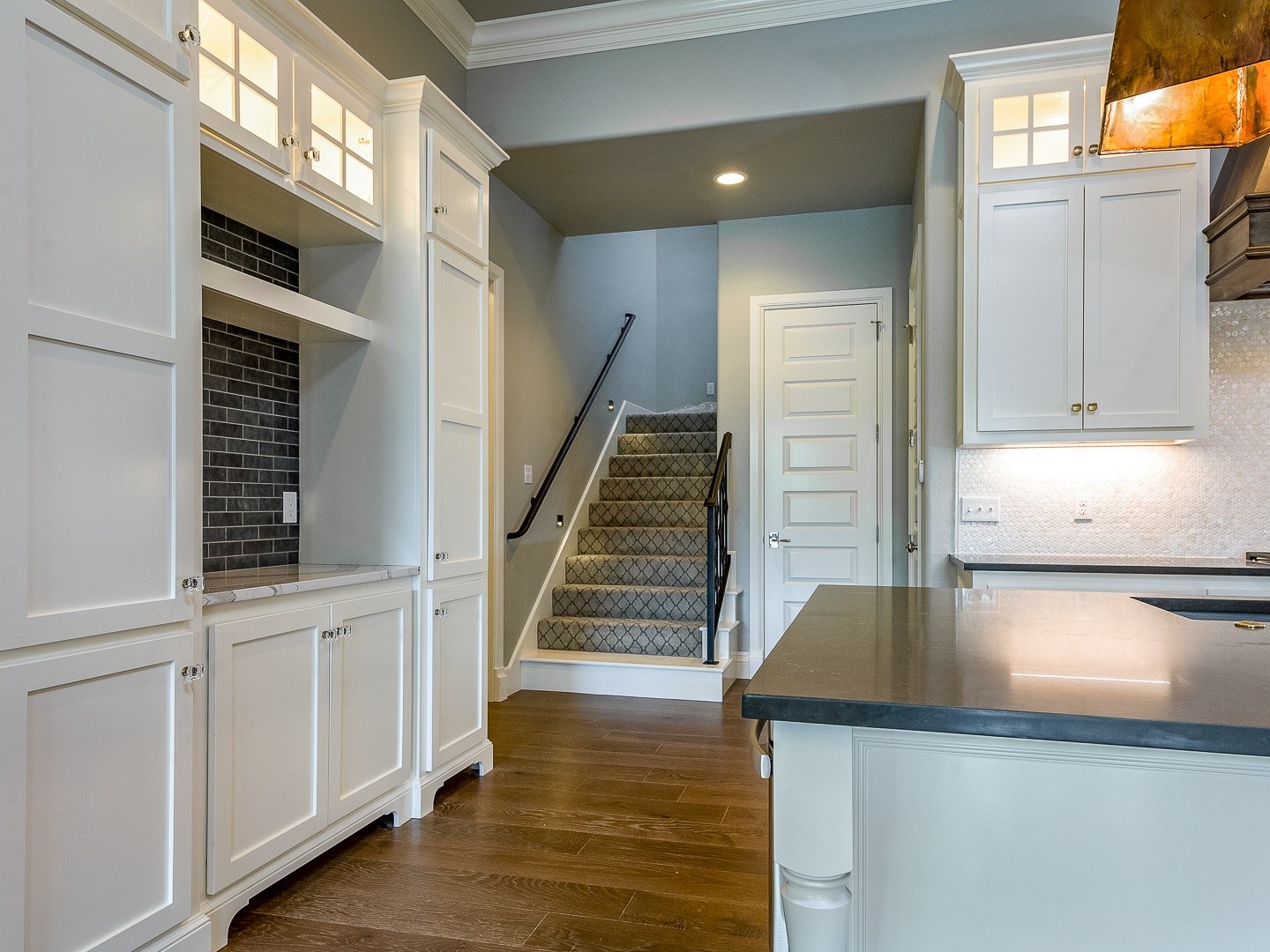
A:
<box><xmin>706</xmin><ymin>381</ymin><xmax>716</xmax><ymax>396</ymax></box>
<box><xmin>1074</xmin><ymin>491</ymin><xmax>1094</xmax><ymax>523</ymax></box>
<box><xmin>282</xmin><ymin>492</ymin><xmax>298</xmax><ymax>525</ymax></box>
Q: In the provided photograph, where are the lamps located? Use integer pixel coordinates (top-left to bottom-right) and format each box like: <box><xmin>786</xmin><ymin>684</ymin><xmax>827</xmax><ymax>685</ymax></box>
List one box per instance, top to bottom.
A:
<box><xmin>1096</xmin><ymin>0</ymin><xmax>1270</xmax><ymax>157</ymax></box>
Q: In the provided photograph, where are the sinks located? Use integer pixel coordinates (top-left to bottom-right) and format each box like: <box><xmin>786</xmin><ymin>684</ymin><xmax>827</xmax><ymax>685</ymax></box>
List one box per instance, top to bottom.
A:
<box><xmin>1166</xmin><ymin>610</ymin><xmax>1270</xmax><ymax>623</ymax></box>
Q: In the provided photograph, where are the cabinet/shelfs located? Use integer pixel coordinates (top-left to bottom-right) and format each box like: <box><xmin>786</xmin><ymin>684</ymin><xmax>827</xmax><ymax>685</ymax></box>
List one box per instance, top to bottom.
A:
<box><xmin>0</xmin><ymin>0</ymin><xmax>206</xmax><ymax>654</ymax></box>
<box><xmin>55</xmin><ymin>0</ymin><xmax>202</xmax><ymax>81</ymax></box>
<box><xmin>200</xmin><ymin>0</ymin><xmax>384</xmax><ymax>250</ymax></box>
<box><xmin>940</xmin><ymin>33</ymin><xmax>1210</xmax><ymax>185</ymax></box>
<box><xmin>426</xmin><ymin>127</ymin><xmax>492</xmax><ymax>268</ymax></box>
<box><xmin>953</xmin><ymin>167</ymin><xmax>1211</xmax><ymax>449</ymax></box>
<box><xmin>425</xmin><ymin>239</ymin><xmax>488</xmax><ymax>583</ymax></box>
<box><xmin>415</xmin><ymin>575</ymin><xmax>494</xmax><ymax>814</ymax></box>
<box><xmin>195</xmin><ymin>578</ymin><xmax>416</xmax><ymax>951</ymax></box>
<box><xmin>0</xmin><ymin>625</ymin><xmax>196</xmax><ymax>952</ymax></box>
